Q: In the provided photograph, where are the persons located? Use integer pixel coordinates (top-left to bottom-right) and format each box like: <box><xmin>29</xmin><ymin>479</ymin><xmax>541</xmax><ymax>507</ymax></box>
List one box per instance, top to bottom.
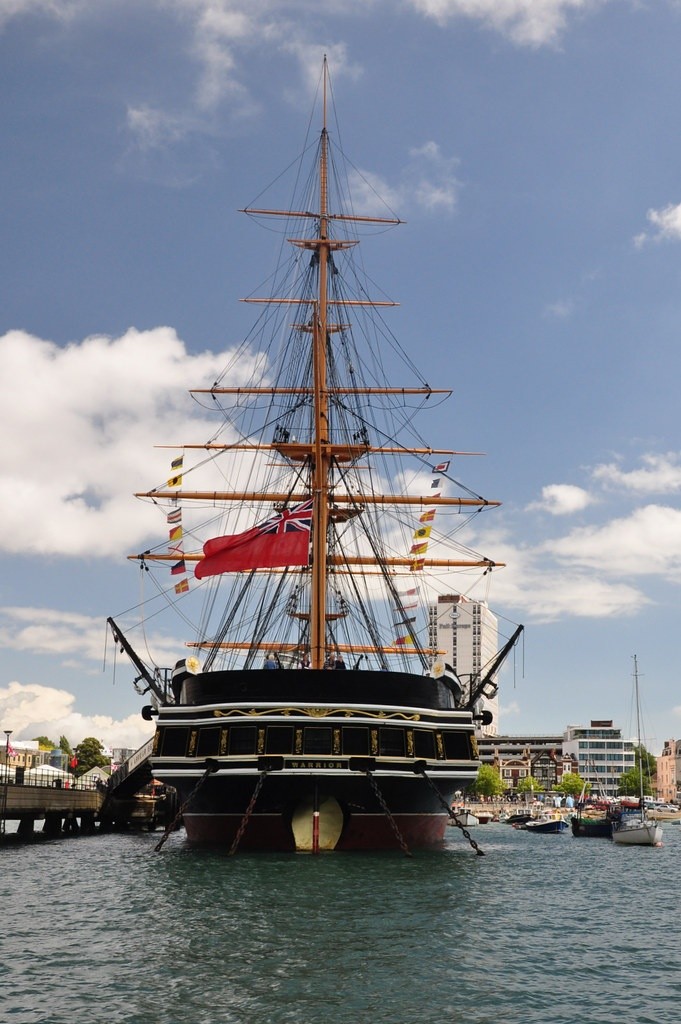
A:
<box><xmin>262</xmin><ymin>649</ymin><xmax>346</xmax><ymax>670</ymax></box>
<box><xmin>93</xmin><ymin>777</ymin><xmax>102</xmax><ymax>789</ymax></box>
<box><xmin>456</xmin><ymin>792</ymin><xmax>574</xmax><ymax>807</ymax></box>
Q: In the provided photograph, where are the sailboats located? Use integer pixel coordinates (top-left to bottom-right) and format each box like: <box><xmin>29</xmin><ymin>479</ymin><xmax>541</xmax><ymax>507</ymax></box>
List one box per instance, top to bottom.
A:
<box><xmin>108</xmin><ymin>51</ymin><xmax>527</xmax><ymax>856</ymax></box>
<box><xmin>612</xmin><ymin>654</ymin><xmax>665</xmax><ymax>846</ymax></box>
<box><xmin>571</xmin><ymin>728</ymin><xmax>615</xmax><ymax>837</ymax></box>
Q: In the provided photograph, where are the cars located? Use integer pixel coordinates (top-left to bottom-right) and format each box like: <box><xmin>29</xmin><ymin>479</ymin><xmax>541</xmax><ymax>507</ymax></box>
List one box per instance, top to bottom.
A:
<box><xmin>652</xmin><ymin>804</ymin><xmax>678</xmax><ymax>812</ymax></box>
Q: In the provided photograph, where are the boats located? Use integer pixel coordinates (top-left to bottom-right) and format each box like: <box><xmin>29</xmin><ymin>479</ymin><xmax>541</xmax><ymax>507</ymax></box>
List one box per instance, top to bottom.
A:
<box><xmin>447</xmin><ymin>800</ymin><xmax>494</xmax><ymax>828</ymax></box>
<box><xmin>489</xmin><ymin>802</ymin><xmax>528</xmax><ymax>823</ymax></box>
<box><xmin>523</xmin><ymin>807</ymin><xmax>569</xmax><ymax>833</ymax></box>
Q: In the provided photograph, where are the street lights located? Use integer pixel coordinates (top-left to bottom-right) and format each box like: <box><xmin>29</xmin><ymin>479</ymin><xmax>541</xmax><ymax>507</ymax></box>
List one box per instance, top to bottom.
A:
<box><xmin>3</xmin><ymin>730</ymin><xmax>13</xmax><ymax>784</ymax></box>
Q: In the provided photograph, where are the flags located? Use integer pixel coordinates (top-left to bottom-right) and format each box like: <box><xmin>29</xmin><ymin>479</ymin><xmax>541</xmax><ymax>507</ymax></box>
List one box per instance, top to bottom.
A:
<box><xmin>167</xmin><ymin>454</ymin><xmax>190</xmax><ymax>594</ymax></box>
<box><xmin>392</xmin><ymin>460</ymin><xmax>450</xmax><ymax>644</ymax></box>
<box><xmin>195</xmin><ymin>495</ymin><xmax>314</xmax><ymax>581</ymax></box>
<box><xmin>429</xmin><ymin>653</ymin><xmax>446</xmax><ymax>680</ymax></box>
